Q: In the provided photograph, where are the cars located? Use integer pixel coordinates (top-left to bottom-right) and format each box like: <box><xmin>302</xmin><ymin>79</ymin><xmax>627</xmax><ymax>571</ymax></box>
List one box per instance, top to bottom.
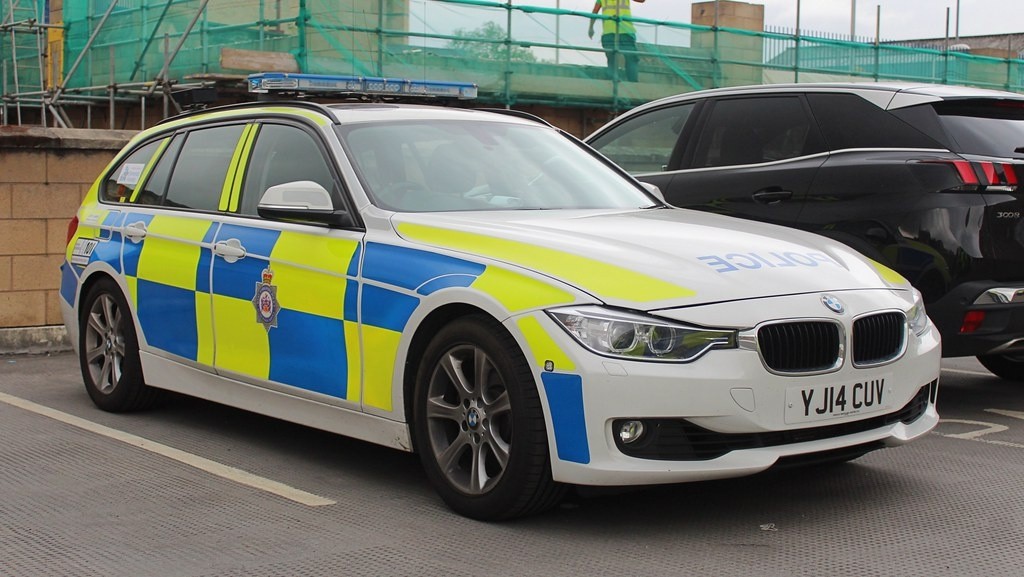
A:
<box><xmin>61</xmin><ymin>71</ymin><xmax>944</xmax><ymax>525</ymax></box>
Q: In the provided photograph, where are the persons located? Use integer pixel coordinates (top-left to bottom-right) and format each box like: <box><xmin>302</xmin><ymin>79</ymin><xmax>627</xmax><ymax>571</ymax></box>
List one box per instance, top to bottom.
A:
<box><xmin>588</xmin><ymin>0</ymin><xmax>646</xmax><ymax>82</ymax></box>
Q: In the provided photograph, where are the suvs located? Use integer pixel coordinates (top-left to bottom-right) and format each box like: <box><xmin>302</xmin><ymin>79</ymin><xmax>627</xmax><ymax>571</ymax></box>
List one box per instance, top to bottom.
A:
<box><xmin>458</xmin><ymin>79</ymin><xmax>1023</xmax><ymax>389</ymax></box>
